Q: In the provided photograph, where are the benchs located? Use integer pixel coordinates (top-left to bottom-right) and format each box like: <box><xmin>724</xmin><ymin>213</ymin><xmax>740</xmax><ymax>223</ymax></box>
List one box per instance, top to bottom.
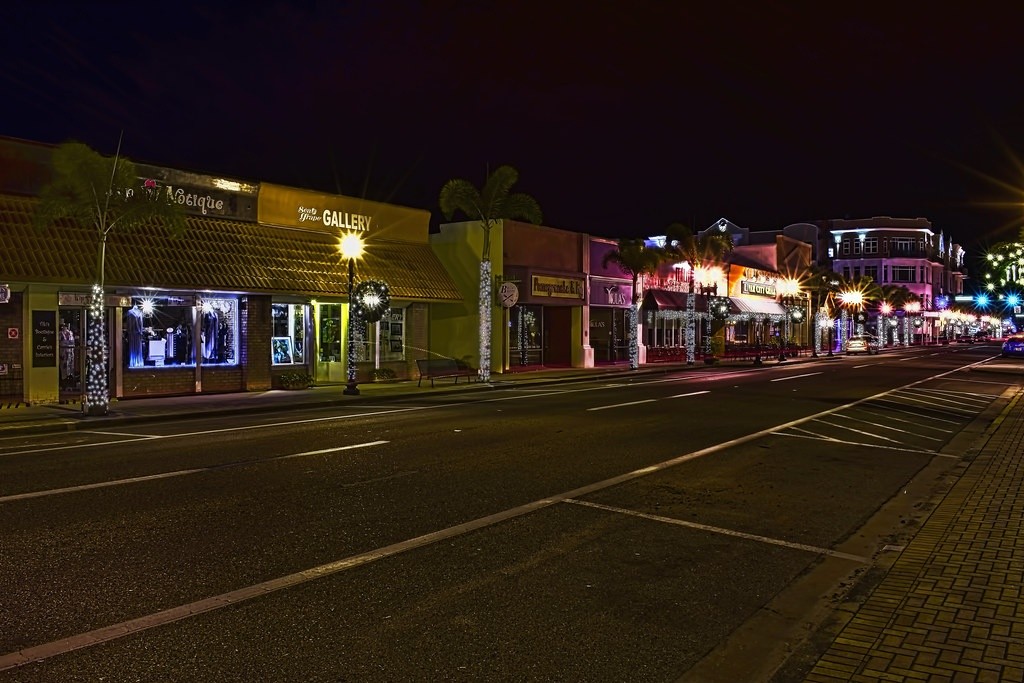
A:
<box><xmin>415</xmin><ymin>359</ymin><xmax>479</xmax><ymax>387</ymax></box>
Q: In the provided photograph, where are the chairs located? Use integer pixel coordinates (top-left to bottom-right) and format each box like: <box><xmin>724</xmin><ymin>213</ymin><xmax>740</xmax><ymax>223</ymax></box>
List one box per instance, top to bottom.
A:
<box><xmin>725</xmin><ymin>342</ymin><xmax>808</xmax><ymax>364</ymax></box>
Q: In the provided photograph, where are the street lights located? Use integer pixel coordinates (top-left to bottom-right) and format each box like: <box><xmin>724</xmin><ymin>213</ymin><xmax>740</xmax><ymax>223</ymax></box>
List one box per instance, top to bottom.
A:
<box><xmin>343</xmin><ymin>237</ymin><xmax>360</xmax><ymax>396</ymax></box>
<box><xmin>695</xmin><ymin>269</ymin><xmax>722</xmax><ymax>363</ymax></box>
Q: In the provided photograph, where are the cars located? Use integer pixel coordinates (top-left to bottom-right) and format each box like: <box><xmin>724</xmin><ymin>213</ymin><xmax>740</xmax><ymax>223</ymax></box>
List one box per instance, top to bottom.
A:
<box><xmin>974</xmin><ymin>332</ymin><xmax>992</xmax><ymax>341</ymax></box>
<box><xmin>845</xmin><ymin>335</ymin><xmax>879</xmax><ymax>357</ymax></box>
<box><xmin>1002</xmin><ymin>336</ymin><xmax>1024</xmax><ymax>358</ymax></box>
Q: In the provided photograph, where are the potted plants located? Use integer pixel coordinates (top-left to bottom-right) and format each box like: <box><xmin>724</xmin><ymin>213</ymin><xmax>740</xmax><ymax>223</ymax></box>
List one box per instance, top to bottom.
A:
<box><xmin>368</xmin><ymin>367</ymin><xmax>397</xmax><ymax>383</ymax></box>
<box><xmin>280</xmin><ymin>371</ymin><xmax>316</xmax><ymax>390</ymax></box>
